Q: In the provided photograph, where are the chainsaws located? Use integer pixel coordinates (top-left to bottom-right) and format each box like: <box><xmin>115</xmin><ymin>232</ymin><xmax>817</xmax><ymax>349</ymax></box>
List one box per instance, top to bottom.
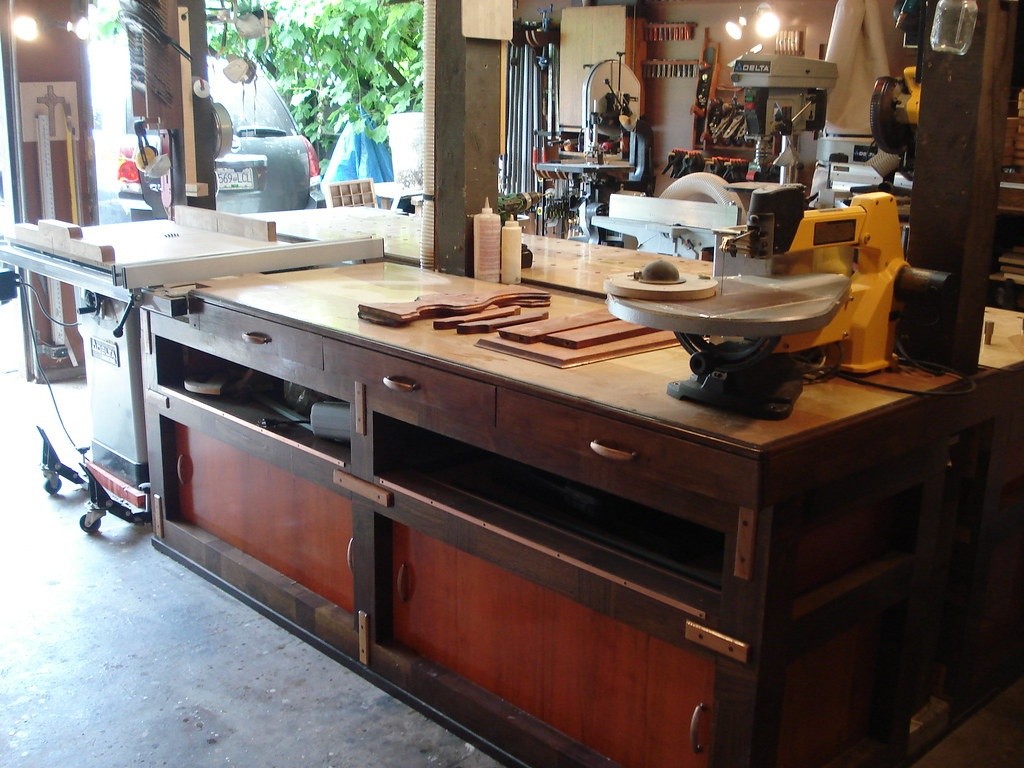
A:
<box><xmin>602</xmin><ymin>46</ymin><xmax>960</xmax><ymax>422</ymax></box>
<box><xmin>538</xmin><ymin>58</ymin><xmax>654</xmax><ymax>245</ymax></box>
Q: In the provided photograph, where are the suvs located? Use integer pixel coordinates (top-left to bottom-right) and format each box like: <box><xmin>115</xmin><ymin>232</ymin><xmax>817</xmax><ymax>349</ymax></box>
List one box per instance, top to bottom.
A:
<box><xmin>90</xmin><ymin>57</ymin><xmax>328</xmax><ymax>225</ymax></box>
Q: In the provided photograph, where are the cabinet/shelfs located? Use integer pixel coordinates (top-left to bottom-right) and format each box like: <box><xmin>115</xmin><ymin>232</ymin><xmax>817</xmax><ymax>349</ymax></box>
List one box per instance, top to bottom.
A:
<box><xmin>240</xmin><ymin>203</ymin><xmax>1024</xmax><ymax>685</ymax></box>
<box><xmin>137</xmin><ymin>258</ymin><xmax>1001</xmax><ymax>768</ymax></box>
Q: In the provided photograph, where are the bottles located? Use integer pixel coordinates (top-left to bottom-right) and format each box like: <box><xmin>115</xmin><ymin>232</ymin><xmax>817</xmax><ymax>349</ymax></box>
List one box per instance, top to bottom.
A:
<box><xmin>930</xmin><ymin>0</ymin><xmax>978</xmax><ymax>56</ymax></box>
<box><xmin>473</xmin><ymin>196</ymin><xmax>500</xmax><ymax>283</ymax></box>
<box><xmin>502</xmin><ymin>213</ymin><xmax>522</xmax><ymax>284</ymax></box>
<box><xmin>522</xmin><ymin>244</ymin><xmax>532</xmax><ymax>267</ymax></box>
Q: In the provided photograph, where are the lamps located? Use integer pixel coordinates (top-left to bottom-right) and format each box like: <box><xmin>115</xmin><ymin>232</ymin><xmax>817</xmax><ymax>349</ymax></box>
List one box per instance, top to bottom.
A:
<box><xmin>724</xmin><ymin>3</ymin><xmax>780</xmax><ymax>40</ymax></box>
<box><xmin>236</xmin><ymin>8</ymin><xmax>273</xmax><ymax>39</ymax></box>
<box><xmin>222</xmin><ymin>51</ymin><xmax>259</xmax><ymax>85</ymax></box>
